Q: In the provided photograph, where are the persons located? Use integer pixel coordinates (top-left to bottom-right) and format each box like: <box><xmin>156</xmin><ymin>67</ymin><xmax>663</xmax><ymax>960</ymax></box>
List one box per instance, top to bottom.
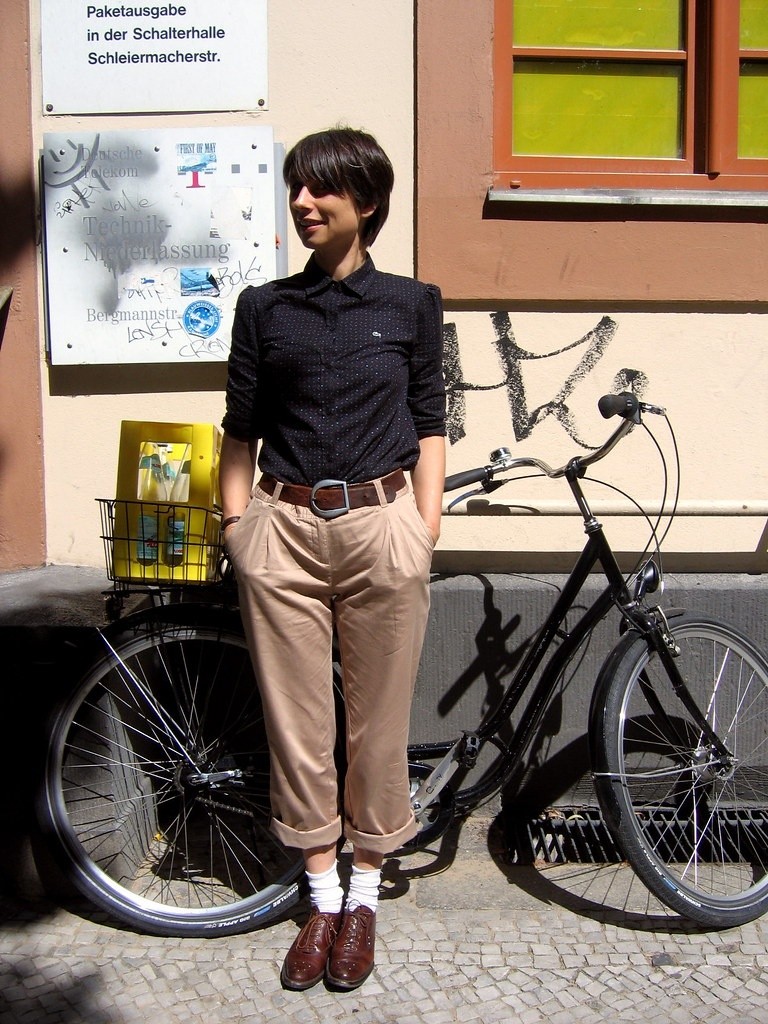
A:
<box><xmin>217</xmin><ymin>128</ymin><xmax>448</xmax><ymax>990</ymax></box>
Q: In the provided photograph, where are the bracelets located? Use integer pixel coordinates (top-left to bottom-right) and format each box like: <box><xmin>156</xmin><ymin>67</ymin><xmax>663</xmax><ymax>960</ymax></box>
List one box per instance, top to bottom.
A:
<box><xmin>220</xmin><ymin>516</ymin><xmax>240</xmax><ymax>530</ymax></box>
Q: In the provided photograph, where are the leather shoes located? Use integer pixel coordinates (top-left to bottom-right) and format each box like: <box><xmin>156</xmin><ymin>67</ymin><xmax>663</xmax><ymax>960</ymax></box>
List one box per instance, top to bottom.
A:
<box><xmin>325</xmin><ymin>899</ymin><xmax>376</xmax><ymax>989</ymax></box>
<box><xmin>279</xmin><ymin>904</ymin><xmax>344</xmax><ymax>992</ymax></box>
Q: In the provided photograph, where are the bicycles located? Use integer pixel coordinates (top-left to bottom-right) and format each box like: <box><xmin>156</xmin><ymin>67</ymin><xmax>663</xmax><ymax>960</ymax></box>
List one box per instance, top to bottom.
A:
<box><xmin>39</xmin><ymin>391</ymin><xmax>767</xmax><ymax>938</ymax></box>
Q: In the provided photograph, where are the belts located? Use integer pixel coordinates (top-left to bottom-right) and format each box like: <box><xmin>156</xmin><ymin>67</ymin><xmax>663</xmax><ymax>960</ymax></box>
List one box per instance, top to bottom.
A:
<box><xmin>259</xmin><ymin>471</ymin><xmax>406</xmax><ymax>518</ymax></box>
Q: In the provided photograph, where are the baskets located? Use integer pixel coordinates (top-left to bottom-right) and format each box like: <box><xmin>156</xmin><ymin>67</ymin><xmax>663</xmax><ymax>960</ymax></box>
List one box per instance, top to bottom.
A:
<box><xmin>95</xmin><ymin>498</ymin><xmax>224</xmax><ymax>587</ymax></box>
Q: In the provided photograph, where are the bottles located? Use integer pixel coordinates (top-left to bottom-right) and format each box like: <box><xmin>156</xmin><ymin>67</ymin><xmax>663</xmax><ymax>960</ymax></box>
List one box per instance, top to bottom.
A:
<box><xmin>137</xmin><ymin>440</ymin><xmax>192</xmax><ymax>567</ymax></box>
